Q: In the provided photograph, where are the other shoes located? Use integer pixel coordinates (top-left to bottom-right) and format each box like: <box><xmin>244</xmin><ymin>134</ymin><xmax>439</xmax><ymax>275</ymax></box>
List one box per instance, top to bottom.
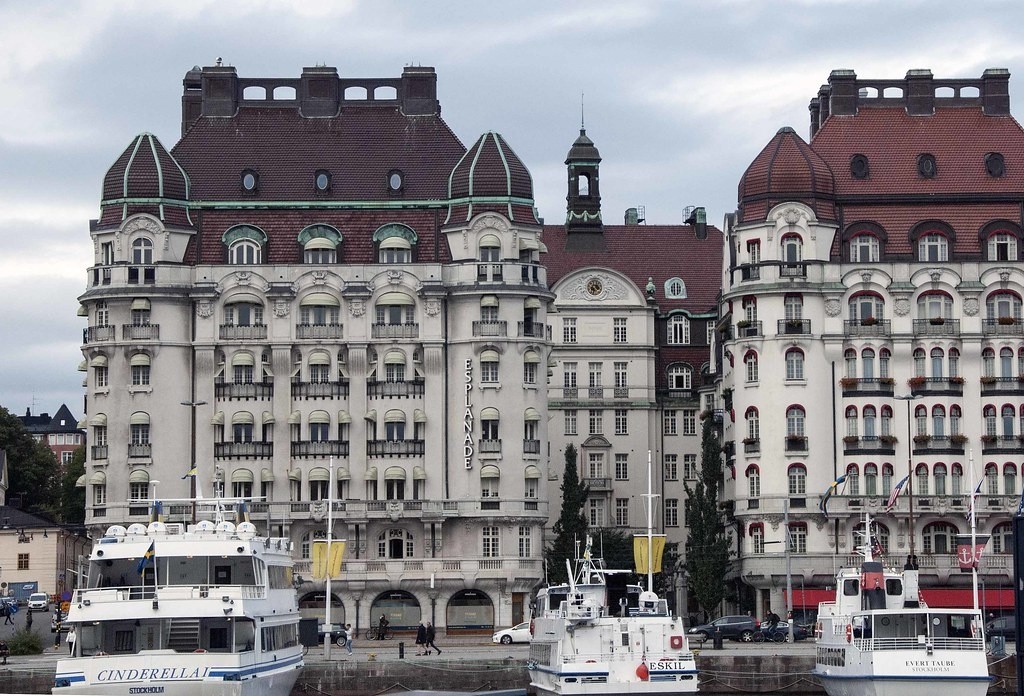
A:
<box><xmin>425</xmin><ymin>650</ymin><xmax>428</xmax><ymax>655</ymax></box>
<box><xmin>438</xmin><ymin>650</ymin><xmax>441</xmax><ymax>655</ymax></box>
<box><xmin>429</xmin><ymin>651</ymin><xmax>431</xmax><ymax>655</ymax></box>
<box><xmin>348</xmin><ymin>653</ymin><xmax>352</xmax><ymax>655</ymax></box>
<box><xmin>416</xmin><ymin>654</ymin><xmax>421</xmax><ymax>656</ymax></box>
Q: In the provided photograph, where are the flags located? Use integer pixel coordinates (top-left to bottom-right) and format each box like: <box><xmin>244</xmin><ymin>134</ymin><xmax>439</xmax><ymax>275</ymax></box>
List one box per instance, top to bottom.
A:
<box><xmin>819</xmin><ymin>474</ymin><xmax>847</xmax><ymax>516</ymax></box>
<box><xmin>888</xmin><ymin>476</ymin><xmax>908</xmax><ymax>508</ymax></box>
<box><xmin>965</xmin><ymin>477</ymin><xmax>984</xmax><ymax>524</ymax></box>
<box><xmin>136</xmin><ymin>542</ymin><xmax>154</xmax><ymax>576</ymax></box>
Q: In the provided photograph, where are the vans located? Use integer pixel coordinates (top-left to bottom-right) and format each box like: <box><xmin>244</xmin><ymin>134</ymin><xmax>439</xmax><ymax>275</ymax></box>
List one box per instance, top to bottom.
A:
<box><xmin>983</xmin><ymin>617</ymin><xmax>1015</xmax><ymax>641</ymax></box>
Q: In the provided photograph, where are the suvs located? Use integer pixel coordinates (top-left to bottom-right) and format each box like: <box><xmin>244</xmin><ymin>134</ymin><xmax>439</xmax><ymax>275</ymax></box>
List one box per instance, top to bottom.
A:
<box><xmin>27</xmin><ymin>593</ymin><xmax>50</xmax><ymax>611</ymax></box>
<box><xmin>689</xmin><ymin>615</ymin><xmax>760</xmax><ymax>643</ymax></box>
<box><xmin>318</xmin><ymin>623</ymin><xmax>348</xmax><ymax>647</ymax></box>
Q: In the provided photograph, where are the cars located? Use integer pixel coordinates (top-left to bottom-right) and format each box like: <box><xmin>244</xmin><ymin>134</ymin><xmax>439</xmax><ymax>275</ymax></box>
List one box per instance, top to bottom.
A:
<box><xmin>0</xmin><ymin>597</ymin><xmax>18</xmax><ymax>616</ymax></box>
<box><xmin>760</xmin><ymin>621</ymin><xmax>807</xmax><ymax>642</ymax></box>
<box><xmin>492</xmin><ymin>622</ymin><xmax>529</xmax><ymax>644</ymax></box>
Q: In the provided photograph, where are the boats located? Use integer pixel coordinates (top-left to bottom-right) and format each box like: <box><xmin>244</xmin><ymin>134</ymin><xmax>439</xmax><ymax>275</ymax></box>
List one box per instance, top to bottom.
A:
<box><xmin>50</xmin><ymin>465</ymin><xmax>318</xmax><ymax>696</ymax></box>
<box><xmin>810</xmin><ymin>513</ymin><xmax>995</xmax><ymax>696</ymax></box>
<box><xmin>526</xmin><ymin>532</ymin><xmax>700</xmax><ymax>696</ymax></box>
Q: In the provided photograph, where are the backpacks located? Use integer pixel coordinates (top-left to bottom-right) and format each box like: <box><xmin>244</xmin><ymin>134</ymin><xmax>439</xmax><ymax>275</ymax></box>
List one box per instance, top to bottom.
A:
<box><xmin>773</xmin><ymin>613</ymin><xmax>780</xmax><ymax>622</ymax></box>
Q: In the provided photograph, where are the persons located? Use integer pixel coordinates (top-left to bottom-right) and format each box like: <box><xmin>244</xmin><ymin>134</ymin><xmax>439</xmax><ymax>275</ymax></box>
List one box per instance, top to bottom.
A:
<box><xmin>4</xmin><ymin>603</ymin><xmax>14</xmax><ymax>625</ymax></box>
<box><xmin>748</xmin><ymin>610</ymin><xmax>752</xmax><ymax>616</ymax></box>
<box><xmin>764</xmin><ymin>610</ymin><xmax>778</xmax><ymax>640</ymax></box>
<box><xmin>376</xmin><ymin>614</ymin><xmax>385</xmax><ymax>640</ymax></box>
<box><xmin>416</xmin><ymin>621</ymin><xmax>442</xmax><ymax>656</ymax></box>
<box><xmin>345</xmin><ymin>624</ymin><xmax>354</xmax><ymax>656</ymax></box>
<box><xmin>66</xmin><ymin>625</ymin><xmax>76</xmax><ymax>655</ymax></box>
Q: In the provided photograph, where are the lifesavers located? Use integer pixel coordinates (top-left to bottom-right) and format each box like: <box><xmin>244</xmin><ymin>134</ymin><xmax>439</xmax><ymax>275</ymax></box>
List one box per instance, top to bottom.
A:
<box><xmin>862</xmin><ymin>572</ymin><xmax>866</xmax><ymax>586</ymax></box>
<box><xmin>918</xmin><ymin>591</ymin><xmax>925</xmax><ymax>602</ymax></box>
<box><xmin>815</xmin><ymin>621</ymin><xmax>818</xmax><ymax>637</ymax></box>
<box><xmin>818</xmin><ymin>622</ymin><xmax>822</xmax><ymax>639</ymax></box>
<box><xmin>530</xmin><ymin>623</ymin><xmax>534</xmax><ymax>634</ymax></box>
<box><xmin>846</xmin><ymin>624</ymin><xmax>851</xmax><ymax>642</ymax></box>
<box><xmin>586</xmin><ymin>660</ymin><xmax>596</xmax><ymax>663</ymax></box>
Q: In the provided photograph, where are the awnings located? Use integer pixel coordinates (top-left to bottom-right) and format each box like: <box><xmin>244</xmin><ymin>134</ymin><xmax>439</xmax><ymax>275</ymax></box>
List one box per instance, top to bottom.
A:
<box><xmin>211</xmin><ymin>292</ymin><xmax>428</xmax><ymax>483</ymax></box>
<box><xmin>480</xmin><ymin>296</ymin><xmax>542</xmax><ymax>478</ymax></box>
<box><xmin>75</xmin><ymin>298</ymin><xmax>150</xmax><ymax>486</ymax></box>
<box><xmin>784</xmin><ymin>590</ymin><xmax>1015</xmax><ymax>609</ymax></box>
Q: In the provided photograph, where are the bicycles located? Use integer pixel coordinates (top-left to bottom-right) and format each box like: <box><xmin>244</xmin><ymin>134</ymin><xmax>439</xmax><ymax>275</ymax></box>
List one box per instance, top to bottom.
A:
<box><xmin>365</xmin><ymin>621</ymin><xmax>394</xmax><ymax>641</ymax></box>
<box><xmin>752</xmin><ymin>622</ymin><xmax>785</xmax><ymax>645</ymax></box>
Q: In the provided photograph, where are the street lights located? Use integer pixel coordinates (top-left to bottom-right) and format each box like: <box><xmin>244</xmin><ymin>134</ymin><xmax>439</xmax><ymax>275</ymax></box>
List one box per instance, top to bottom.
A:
<box><xmin>893</xmin><ymin>393</ymin><xmax>924</xmax><ymax>555</ymax></box>
<box><xmin>180</xmin><ymin>401</ymin><xmax>208</xmax><ymax>524</ymax></box>
